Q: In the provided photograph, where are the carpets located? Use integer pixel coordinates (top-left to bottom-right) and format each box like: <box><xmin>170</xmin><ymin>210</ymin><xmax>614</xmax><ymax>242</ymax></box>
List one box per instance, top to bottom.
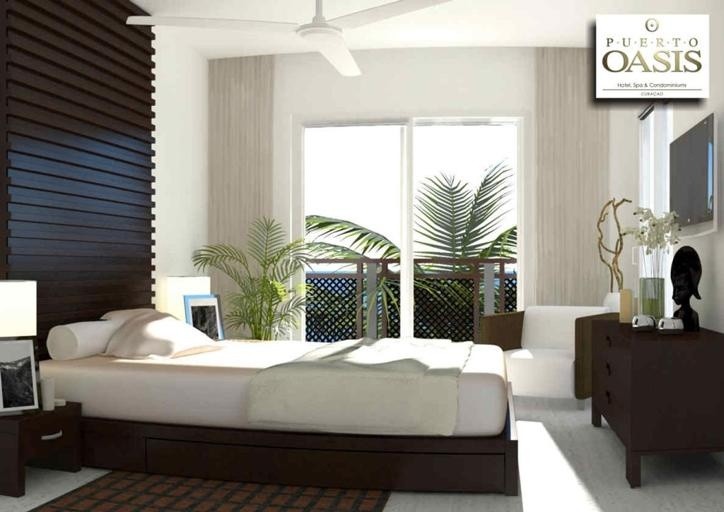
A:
<box><xmin>28</xmin><ymin>471</ymin><xmax>394</xmax><ymax>511</ymax></box>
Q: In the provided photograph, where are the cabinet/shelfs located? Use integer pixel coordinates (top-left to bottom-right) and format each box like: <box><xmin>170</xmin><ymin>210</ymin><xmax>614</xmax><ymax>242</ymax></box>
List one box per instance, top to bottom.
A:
<box><xmin>591</xmin><ymin>319</ymin><xmax>724</xmax><ymax>487</ymax></box>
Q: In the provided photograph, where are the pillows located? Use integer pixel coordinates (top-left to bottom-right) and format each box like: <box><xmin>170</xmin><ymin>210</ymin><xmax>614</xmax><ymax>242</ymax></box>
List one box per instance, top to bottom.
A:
<box><xmin>46</xmin><ymin>307</ymin><xmax>222</xmax><ymax>361</ymax></box>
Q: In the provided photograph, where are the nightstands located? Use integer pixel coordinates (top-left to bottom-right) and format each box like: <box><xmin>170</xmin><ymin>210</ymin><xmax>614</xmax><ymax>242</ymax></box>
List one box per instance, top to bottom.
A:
<box><xmin>0</xmin><ymin>400</ymin><xmax>81</xmax><ymax>497</ymax></box>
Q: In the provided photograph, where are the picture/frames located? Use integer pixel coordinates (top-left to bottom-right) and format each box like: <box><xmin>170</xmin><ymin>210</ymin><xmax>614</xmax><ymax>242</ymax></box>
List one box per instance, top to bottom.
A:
<box><xmin>184</xmin><ymin>293</ymin><xmax>225</xmax><ymax>341</ymax></box>
<box><xmin>0</xmin><ymin>336</ymin><xmax>39</xmax><ymax>415</ymax></box>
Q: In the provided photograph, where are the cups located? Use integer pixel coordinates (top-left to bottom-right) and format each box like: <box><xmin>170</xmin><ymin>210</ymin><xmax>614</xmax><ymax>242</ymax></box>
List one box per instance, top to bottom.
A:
<box><xmin>633</xmin><ymin>315</ymin><xmax>654</xmax><ymax>332</ymax></box>
<box><xmin>658</xmin><ymin>316</ymin><xmax>684</xmax><ymax>336</ymax></box>
<box><xmin>40</xmin><ymin>378</ymin><xmax>56</xmax><ymax>411</ymax></box>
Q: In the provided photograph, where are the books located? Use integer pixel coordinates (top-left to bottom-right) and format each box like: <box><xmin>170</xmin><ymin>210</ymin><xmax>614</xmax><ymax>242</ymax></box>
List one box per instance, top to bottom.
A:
<box><xmin>638</xmin><ymin>277</ymin><xmax>665</xmax><ymax>319</ymax></box>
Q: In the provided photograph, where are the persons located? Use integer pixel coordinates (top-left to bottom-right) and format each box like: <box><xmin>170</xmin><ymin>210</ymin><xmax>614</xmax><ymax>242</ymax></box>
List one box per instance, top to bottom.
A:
<box><xmin>669</xmin><ymin>245</ymin><xmax>704</xmax><ymax>333</ymax></box>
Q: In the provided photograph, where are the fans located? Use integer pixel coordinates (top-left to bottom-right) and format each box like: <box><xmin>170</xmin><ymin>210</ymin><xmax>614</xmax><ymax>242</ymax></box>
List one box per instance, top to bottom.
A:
<box><xmin>126</xmin><ymin>0</ymin><xmax>448</xmax><ymax>76</ymax></box>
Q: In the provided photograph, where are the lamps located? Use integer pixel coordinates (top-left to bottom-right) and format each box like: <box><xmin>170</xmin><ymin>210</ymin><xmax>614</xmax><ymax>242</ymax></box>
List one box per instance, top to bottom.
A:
<box><xmin>168</xmin><ymin>275</ymin><xmax>211</xmax><ymax>321</ymax></box>
<box><xmin>0</xmin><ymin>278</ymin><xmax>38</xmax><ymax>337</ymax></box>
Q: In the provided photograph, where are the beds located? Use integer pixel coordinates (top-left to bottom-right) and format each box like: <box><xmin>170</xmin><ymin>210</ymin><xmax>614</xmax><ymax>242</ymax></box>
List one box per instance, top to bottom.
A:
<box><xmin>36</xmin><ymin>338</ymin><xmax>519</xmax><ymax>497</ymax></box>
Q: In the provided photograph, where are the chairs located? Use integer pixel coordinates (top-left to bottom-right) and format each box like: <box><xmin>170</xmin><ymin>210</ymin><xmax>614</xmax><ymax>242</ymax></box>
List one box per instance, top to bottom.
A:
<box><xmin>478</xmin><ymin>306</ymin><xmax>620</xmax><ymax>411</ymax></box>
